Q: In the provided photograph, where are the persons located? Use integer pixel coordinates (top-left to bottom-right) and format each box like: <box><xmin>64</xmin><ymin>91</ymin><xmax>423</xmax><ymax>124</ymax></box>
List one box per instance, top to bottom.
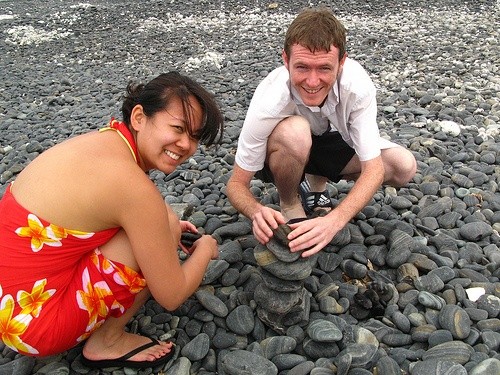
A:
<box><xmin>226</xmin><ymin>7</ymin><xmax>419</xmax><ymax>258</ymax></box>
<box><xmin>0</xmin><ymin>71</ymin><xmax>226</xmax><ymax>370</ymax></box>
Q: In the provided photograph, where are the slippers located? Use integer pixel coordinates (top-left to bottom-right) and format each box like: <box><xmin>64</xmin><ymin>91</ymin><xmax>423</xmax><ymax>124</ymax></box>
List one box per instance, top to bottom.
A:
<box><xmin>299</xmin><ymin>183</ymin><xmax>334</xmax><ymax>215</ymax></box>
<box><xmin>78</xmin><ymin>334</ymin><xmax>175</xmax><ymax>368</ymax></box>
